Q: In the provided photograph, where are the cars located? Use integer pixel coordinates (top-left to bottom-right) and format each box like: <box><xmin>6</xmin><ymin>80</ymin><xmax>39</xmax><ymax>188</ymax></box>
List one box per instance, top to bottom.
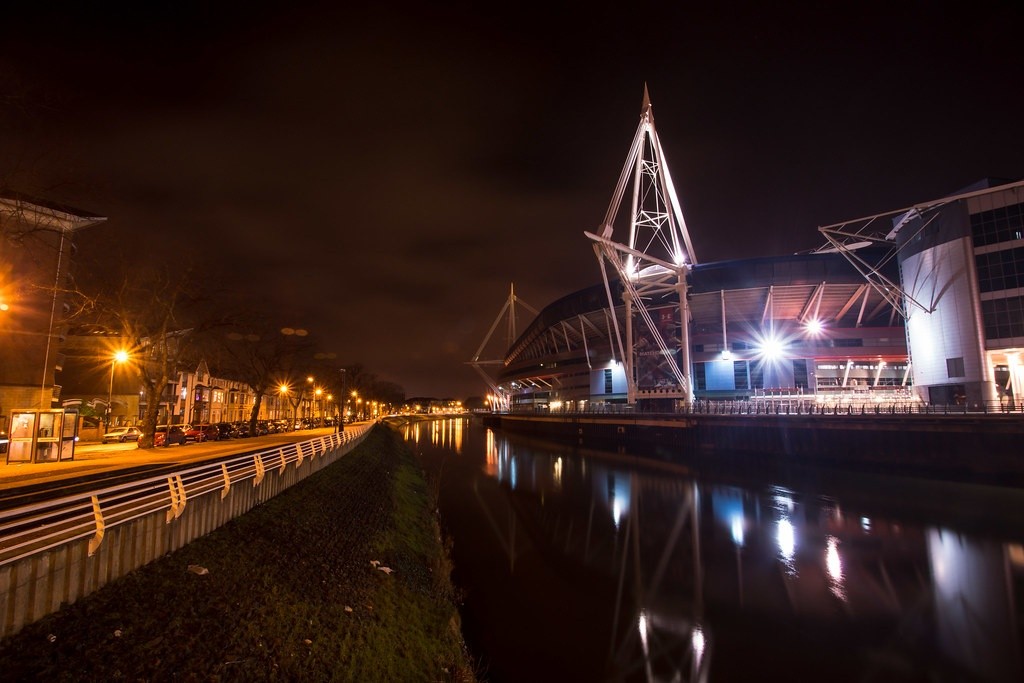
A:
<box><xmin>179</xmin><ymin>415</ymin><xmax>360</xmax><ymax>442</ymax></box>
<box><xmin>137</xmin><ymin>425</ymin><xmax>187</xmax><ymax>449</ymax></box>
<box><xmin>100</xmin><ymin>427</ymin><xmax>144</xmax><ymax>444</ymax></box>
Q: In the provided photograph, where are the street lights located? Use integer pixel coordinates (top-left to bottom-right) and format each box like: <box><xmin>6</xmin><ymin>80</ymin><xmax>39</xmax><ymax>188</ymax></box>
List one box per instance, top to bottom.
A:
<box><xmin>103</xmin><ymin>349</ymin><xmax>130</xmax><ymax>435</ymax></box>
<box><xmin>306</xmin><ymin>376</ymin><xmax>316</xmax><ymax>419</ymax></box>
